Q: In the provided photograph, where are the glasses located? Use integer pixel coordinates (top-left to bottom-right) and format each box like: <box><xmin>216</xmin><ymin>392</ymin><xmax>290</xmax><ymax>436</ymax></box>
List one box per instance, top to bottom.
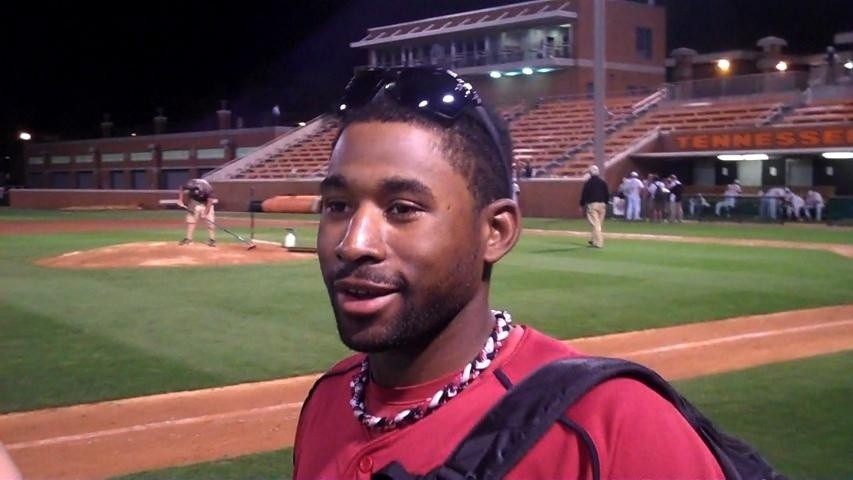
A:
<box><xmin>336</xmin><ymin>65</ymin><xmax>513</xmax><ymax>199</ymax></box>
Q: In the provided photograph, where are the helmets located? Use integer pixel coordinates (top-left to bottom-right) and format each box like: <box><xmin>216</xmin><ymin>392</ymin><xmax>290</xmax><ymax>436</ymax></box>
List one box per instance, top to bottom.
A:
<box><xmin>189</xmin><ymin>186</ymin><xmax>202</xmax><ymax>201</ymax></box>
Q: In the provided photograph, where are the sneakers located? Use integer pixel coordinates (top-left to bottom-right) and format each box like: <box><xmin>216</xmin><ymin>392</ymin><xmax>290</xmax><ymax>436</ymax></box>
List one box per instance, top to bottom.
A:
<box><xmin>179</xmin><ymin>238</ymin><xmax>193</xmax><ymax>247</ymax></box>
<box><xmin>208</xmin><ymin>239</ymin><xmax>215</xmax><ymax>246</ymax></box>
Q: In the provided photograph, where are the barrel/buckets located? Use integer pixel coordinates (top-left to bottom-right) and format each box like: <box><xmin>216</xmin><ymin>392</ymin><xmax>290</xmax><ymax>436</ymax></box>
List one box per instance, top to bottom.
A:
<box><xmin>284</xmin><ymin>228</ymin><xmax>296</xmax><ymax>247</ymax></box>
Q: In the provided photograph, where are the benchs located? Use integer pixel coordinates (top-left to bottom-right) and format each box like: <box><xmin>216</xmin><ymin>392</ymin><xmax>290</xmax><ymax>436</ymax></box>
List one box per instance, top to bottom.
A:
<box><xmin>666</xmin><ymin>93</ymin><xmax>848</xmax><ymax>130</ymax></box>
<box><xmin>234</xmin><ymin>94</ymin><xmax>665</xmax><ymax>177</ymax></box>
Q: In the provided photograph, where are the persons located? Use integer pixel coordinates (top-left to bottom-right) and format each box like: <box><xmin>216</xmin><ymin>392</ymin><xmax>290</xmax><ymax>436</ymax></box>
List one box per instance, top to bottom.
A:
<box><xmin>822</xmin><ymin>46</ymin><xmax>840</xmax><ymax>86</ymax></box>
<box><xmin>292</xmin><ymin>64</ymin><xmax>729</xmax><ymax>480</ymax></box>
<box><xmin>578</xmin><ymin>165</ymin><xmax>609</xmax><ymax>249</ymax></box>
<box><xmin>616</xmin><ymin>169</ymin><xmax>826</xmax><ymax>225</ymax></box>
<box><xmin>176</xmin><ymin>178</ymin><xmax>217</xmax><ymax>248</ymax></box>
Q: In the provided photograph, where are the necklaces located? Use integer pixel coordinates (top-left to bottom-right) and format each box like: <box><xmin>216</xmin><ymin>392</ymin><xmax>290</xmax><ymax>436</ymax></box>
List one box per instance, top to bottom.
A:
<box><xmin>347</xmin><ymin>306</ymin><xmax>513</xmax><ymax>431</ymax></box>
